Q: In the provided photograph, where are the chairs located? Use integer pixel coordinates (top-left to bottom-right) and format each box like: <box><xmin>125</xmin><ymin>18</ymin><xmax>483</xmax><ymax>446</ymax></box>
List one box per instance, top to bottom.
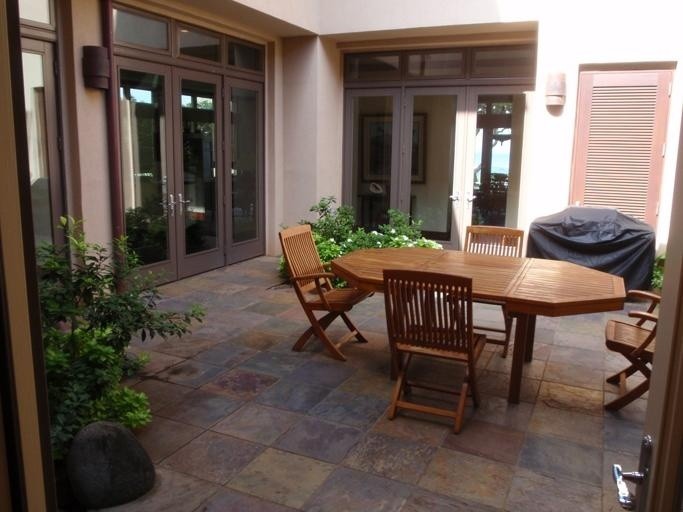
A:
<box><xmin>278</xmin><ymin>224</ymin><xmax>374</xmax><ymax>361</ymax></box>
<box><xmin>446</xmin><ymin>226</ymin><xmax>524</xmax><ymax>359</ymax></box>
<box><xmin>382</xmin><ymin>268</ymin><xmax>486</xmax><ymax>435</ymax></box>
<box><xmin>604</xmin><ymin>289</ymin><xmax>661</xmax><ymax>413</ymax></box>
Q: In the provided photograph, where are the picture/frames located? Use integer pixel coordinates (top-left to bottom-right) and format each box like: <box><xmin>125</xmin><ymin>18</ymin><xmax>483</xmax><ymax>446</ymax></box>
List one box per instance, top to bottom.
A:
<box><xmin>360</xmin><ymin>113</ymin><xmax>428</xmax><ymax>184</ymax></box>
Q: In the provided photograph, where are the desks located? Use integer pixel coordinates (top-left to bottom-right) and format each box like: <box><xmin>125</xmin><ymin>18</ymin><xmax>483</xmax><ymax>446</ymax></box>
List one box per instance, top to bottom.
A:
<box><xmin>357</xmin><ymin>194</ymin><xmax>415</xmax><ymax>233</ymax></box>
<box><xmin>332</xmin><ymin>248</ymin><xmax>626</xmax><ymax>405</ymax></box>
